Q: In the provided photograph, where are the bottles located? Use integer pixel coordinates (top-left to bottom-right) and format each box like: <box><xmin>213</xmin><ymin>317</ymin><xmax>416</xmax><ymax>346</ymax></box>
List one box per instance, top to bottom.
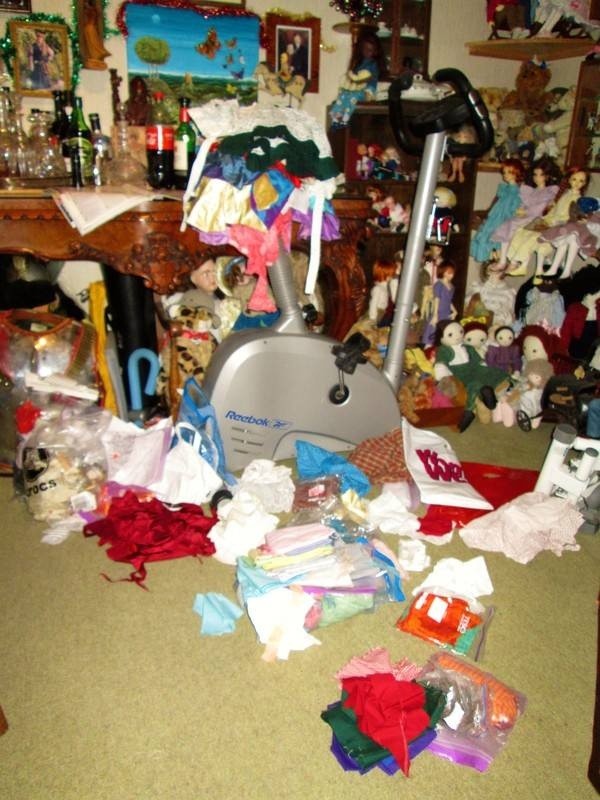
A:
<box><xmin>0</xmin><ymin>48</ymin><xmax>67</xmax><ymax>180</ymax></box>
<box><xmin>47</xmin><ymin>90</ymin><xmax>74</xmax><ymax>178</ymax></box>
<box><xmin>146</xmin><ymin>92</ymin><xmax>175</xmax><ymax>190</ymax></box>
<box><xmin>69</xmin><ymin>97</ymin><xmax>94</xmax><ymax>187</ymax></box>
<box><xmin>175</xmin><ymin>97</ymin><xmax>196</xmax><ymax>190</ymax></box>
<box><xmin>108</xmin><ymin>103</ymin><xmax>147</xmax><ymax>190</ymax></box>
<box><xmin>88</xmin><ymin>113</ymin><xmax>113</xmax><ymax>185</ymax></box>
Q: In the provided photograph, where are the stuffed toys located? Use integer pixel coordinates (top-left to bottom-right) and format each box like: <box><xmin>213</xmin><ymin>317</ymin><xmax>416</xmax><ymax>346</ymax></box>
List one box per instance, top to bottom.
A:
<box><xmin>474</xmin><ymin>54</ymin><xmax>576</xmax><ymax>162</ymax></box>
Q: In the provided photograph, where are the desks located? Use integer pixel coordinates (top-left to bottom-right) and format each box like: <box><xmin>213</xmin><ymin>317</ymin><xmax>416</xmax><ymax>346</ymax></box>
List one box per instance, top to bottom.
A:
<box><xmin>0</xmin><ymin>178</ymin><xmax>374</xmax><ymax>345</ymax></box>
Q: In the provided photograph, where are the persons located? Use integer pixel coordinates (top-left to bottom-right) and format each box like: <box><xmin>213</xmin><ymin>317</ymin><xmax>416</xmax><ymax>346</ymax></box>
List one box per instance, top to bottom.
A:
<box><xmin>485</xmin><ymin>0</ymin><xmax>531</xmax><ymax>40</ymax></box>
<box><xmin>27</xmin><ymin>30</ymin><xmax>54</xmax><ymax>88</ymax></box>
<box><xmin>287</xmin><ymin>33</ymin><xmax>309</xmax><ymax>76</ymax></box>
<box><xmin>328</xmin><ymin>33</ymin><xmax>386</xmax><ymax>130</ymax></box>
<box><xmin>280</xmin><ymin>53</ymin><xmax>295</xmax><ymax>96</ymax></box>
<box><xmin>152</xmin><ymin>127</ymin><xmax>600</xmax><ymax>430</ymax></box>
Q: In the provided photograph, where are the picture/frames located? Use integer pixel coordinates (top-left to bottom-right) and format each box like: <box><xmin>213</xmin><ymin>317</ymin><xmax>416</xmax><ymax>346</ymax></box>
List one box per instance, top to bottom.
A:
<box><xmin>265</xmin><ymin>11</ymin><xmax>321</xmax><ymax>94</ymax></box>
<box><xmin>10</xmin><ymin>20</ymin><xmax>70</xmax><ymax>98</ymax></box>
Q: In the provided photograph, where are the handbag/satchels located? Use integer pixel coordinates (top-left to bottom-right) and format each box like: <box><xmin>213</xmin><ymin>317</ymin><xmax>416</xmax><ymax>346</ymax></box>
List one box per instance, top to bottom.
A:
<box><xmin>23</xmin><ymin>410</ymin><xmax>114</xmax><ymax>521</ymax></box>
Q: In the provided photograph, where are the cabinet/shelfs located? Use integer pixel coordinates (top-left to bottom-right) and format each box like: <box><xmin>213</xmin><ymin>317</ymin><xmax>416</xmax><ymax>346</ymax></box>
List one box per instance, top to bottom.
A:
<box><xmin>465</xmin><ymin>37</ymin><xmax>600</xmax><ymax>176</ymax></box>
<box><xmin>325</xmin><ymin>101</ymin><xmax>503</xmax><ymax>322</ymax></box>
